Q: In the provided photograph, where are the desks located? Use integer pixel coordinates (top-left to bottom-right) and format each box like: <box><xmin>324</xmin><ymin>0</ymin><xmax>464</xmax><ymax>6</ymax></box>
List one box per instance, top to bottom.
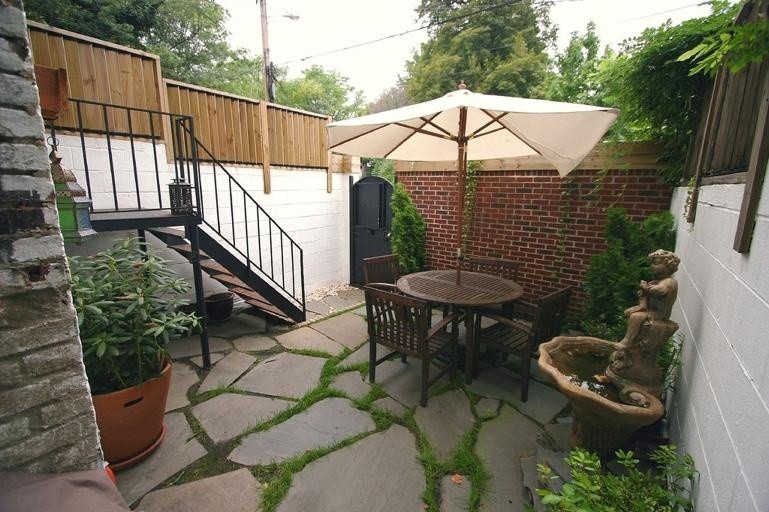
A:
<box><xmin>396</xmin><ymin>271</ymin><xmax>523</xmax><ymax>344</ymax></box>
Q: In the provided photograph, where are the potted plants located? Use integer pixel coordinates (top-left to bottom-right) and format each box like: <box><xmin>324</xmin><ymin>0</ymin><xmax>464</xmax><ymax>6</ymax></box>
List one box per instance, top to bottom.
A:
<box><xmin>66</xmin><ymin>233</ymin><xmax>202</xmax><ymax>471</ymax></box>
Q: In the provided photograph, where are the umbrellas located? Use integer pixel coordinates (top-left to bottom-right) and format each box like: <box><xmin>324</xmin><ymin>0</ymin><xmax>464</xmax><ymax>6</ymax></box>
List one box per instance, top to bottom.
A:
<box><xmin>326</xmin><ymin>89</ymin><xmax>622</xmax><ymax>284</ymax></box>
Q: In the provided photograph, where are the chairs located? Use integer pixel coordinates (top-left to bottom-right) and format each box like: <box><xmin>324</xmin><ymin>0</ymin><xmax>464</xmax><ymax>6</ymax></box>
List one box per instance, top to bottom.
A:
<box><xmin>362</xmin><ymin>253</ymin><xmax>449</xmax><ymax>330</ymax></box>
<box><xmin>458</xmin><ymin>255</ymin><xmax>519</xmax><ymax>320</ymax></box>
<box><xmin>461</xmin><ymin>285</ymin><xmax>572</xmax><ymax>403</ymax></box>
<box><xmin>363</xmin><ymin>286</ymin><xmax>464</xmax><ymax>408</ymax></box>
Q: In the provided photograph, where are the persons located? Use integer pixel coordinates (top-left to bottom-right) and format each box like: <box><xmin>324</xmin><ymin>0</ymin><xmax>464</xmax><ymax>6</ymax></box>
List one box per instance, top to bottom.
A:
<box><xmin>614</xmin><ymin>247</ymin><xmax>680</xmax><ymax>351</ymax></box>
